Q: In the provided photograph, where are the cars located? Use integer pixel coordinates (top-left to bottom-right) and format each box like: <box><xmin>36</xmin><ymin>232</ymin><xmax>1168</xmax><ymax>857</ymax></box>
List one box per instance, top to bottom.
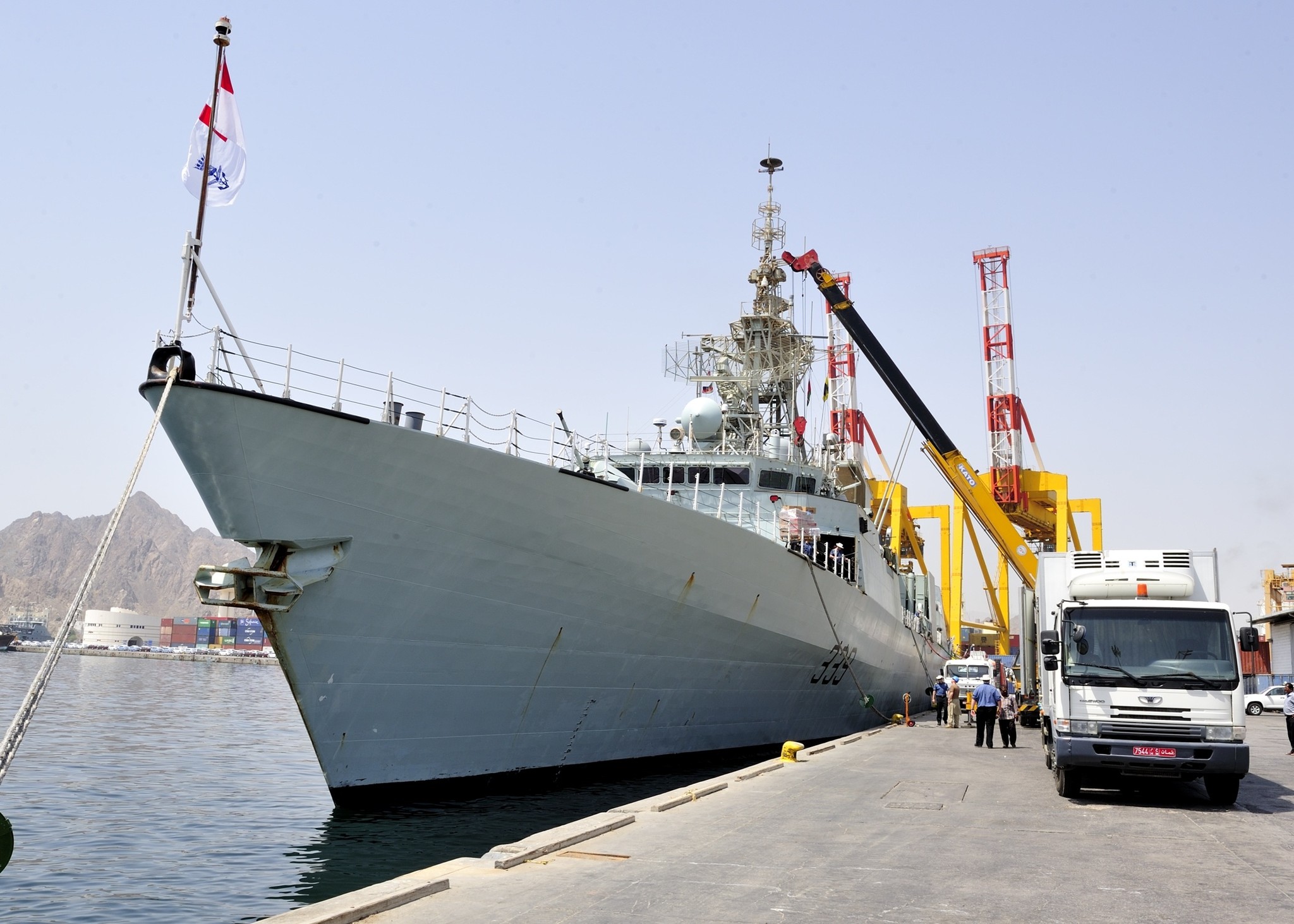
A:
<box><xmin>8</xmin><ymin>638</ymin><xmax>277</xmax><ymax>658</ymax></box>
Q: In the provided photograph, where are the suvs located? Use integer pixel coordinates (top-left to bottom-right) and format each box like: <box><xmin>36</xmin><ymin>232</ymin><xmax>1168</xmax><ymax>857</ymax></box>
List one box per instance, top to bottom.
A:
<box><xmin>1242</xmin><ymin>684</ymin><xmax>1294</xmax><ymax>715</ymax></box>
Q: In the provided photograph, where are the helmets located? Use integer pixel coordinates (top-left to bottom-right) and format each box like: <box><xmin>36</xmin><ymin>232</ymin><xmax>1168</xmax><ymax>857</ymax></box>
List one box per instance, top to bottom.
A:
<box><xmin>953</xmin><ymin>676</ymin><xmax>959</xmax><ymax>683</ymax></box>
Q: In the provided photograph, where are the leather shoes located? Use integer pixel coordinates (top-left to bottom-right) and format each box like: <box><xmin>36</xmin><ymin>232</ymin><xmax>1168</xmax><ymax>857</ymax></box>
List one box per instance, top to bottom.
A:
<box><xmin>944</xmin><ymin>720</ymin><xmax>947</xmax><ymax>724</ymax></box>
<box><xmin>938</xmin><ymin>721</ymin><xmax>941</xmax><ymax>725</ymax></box>
<box><xmin>975</xmin><ymin>743</ymin><xmax>982</xmax><ymax>747</ymax></box>
<box><xmin>988</xmin><ymin>746</ymin><xmax>993</xmax><ymax>748</ymax></box>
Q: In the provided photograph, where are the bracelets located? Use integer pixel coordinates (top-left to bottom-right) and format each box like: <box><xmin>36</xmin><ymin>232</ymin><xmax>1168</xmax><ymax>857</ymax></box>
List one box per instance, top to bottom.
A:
<box><xmin>971</xmin><ymin>709</ymin><xmax>974</xmax><ymax>710</ymax></box>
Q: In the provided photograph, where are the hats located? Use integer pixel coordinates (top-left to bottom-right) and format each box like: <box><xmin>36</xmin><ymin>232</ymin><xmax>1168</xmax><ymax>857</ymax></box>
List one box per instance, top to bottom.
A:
<box><xmin>836</xmin><ymin>542</ymin><xmax>844</xmax><ymax>549</ymax></box>
<box><xmin>981</xmin><ymin>674</ymin><xmax>992</xmax><ymax>680</ymax></box>
<box><xmin>936</xmin><ymin>675</ymin><xmax>944</xmax><ymax>679</ymax></box>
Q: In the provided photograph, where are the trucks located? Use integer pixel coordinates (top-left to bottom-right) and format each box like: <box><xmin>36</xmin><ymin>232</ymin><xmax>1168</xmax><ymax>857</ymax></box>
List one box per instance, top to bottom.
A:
<box><xmin>1035</xmin><ymin>547</ymin><xmax>1250</xmax><ymax>809</ymax></box>
<box><xmin>943</xmin><ymin>651</ymin><xmax>997</xmax><ymax>713</ymax></box>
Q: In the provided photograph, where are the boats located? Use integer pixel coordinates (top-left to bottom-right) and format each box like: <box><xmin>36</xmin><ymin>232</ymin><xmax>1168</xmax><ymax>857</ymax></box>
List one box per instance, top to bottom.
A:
<box><xmin>132</xmin><ymin>17</ymin><xmax>952</xmax><ymax>816</ymax></box>
<box><xmin>0</xmin><ymin>633</ymin><xmax>17</xmax><ymax>652</ymax></box>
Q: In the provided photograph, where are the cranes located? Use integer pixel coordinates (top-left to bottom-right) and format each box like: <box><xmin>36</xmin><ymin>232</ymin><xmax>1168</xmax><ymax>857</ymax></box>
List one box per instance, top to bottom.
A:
<box><xmin>782</xmin><ymin>242</ymin><xmax>1103</xmax><ymax>728</ymax></box>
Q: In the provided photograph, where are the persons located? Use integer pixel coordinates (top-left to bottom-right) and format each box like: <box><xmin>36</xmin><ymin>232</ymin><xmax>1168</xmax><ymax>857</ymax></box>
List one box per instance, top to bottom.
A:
<box><xmin>797</xmin><ymin>537</ymin><xmax>814</xmax><ymax>559</ymax></box>
<box><xmin>829</xmin><ymin>543</ymin><xmax>849</xmax><ymax>577</ymax></box>
<box><xmin>970</xmin><ymin>674</ymin><xmax>1002</xmax><ymax>748</ymax></box>
<box><xmin>932</xmin><ymin>675</ymin><xmax>949</xmax><ymax>725</ymax></box>
<box><xmin>945</xmin><ymin>676</ymin><xmax>962</xmax><ymax>729</ymax></box>
<box><xmin>1282</xmin><ymin>683</ymin><xmax>1294</xmax><ymax>756</ymax></box>
<box><xmin>996</xmin><ymin>685</ymin><xmax>1019</xmax><ymax>748</ymax></box>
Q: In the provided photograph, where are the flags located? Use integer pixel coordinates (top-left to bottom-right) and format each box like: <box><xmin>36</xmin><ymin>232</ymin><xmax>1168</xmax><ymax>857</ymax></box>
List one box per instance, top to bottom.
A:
<box><xmin>180</xmin><ymin>48</ymin><xmax>248</xmax><ymax>209</ymax></box>
<box><xmin>822</xmin><ymin>377</ymin><xmax>828</xmax><ymax>401</ymax></box>
<box><xmin>807</xmin><ymin>379</ymin><xmax>812</xmax><ymax>406</ymax></box>
<box><xmin>702</xmin><ymin>382</ymin><xmax>714</xmax><ymax>393</ymax></box>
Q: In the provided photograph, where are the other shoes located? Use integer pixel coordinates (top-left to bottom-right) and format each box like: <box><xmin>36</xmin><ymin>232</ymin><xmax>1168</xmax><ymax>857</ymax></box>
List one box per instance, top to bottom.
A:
<box><xmin>945</xmin><ymin>725</ymin><xmax>952</xmax><ymax>728</ymax></box>
<box><xmin>952</xmin><ymin>725</ymin><xmax>959</xmax><ymax>728</ymax></box>
<box><xmin>1010</xmin><ymin>743</ymin><xmax>1017</xmax><ymax>748</ymax></box>
<box><xmin>1003</xmin><ymin>745</ymin><xmax>1009</xmax><ymax>748</ymax></box>
<box><xmin>1286</xmin><ymin>750</ymin><xmax>1293</xmax><ymax>755</ymax></box>
<box><xmin>1291</xmin><ymin>753</ymin><xmax>1294</xmax><ymax>756</ymax></box>
